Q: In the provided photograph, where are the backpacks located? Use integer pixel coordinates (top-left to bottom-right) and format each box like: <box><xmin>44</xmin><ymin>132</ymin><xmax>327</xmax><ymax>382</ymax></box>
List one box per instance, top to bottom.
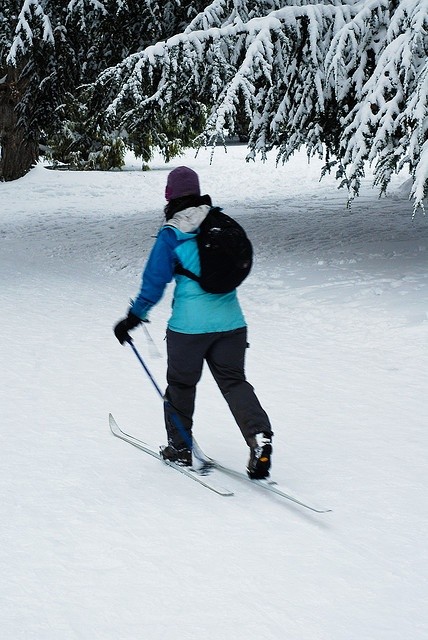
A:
<box><xmin>173</xmin><ymin>207</ymin><xmax>253</xmax><ymax>294</ymax></box>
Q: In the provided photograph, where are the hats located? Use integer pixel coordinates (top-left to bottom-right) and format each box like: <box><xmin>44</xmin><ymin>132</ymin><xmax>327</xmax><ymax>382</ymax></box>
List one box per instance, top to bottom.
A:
<box><xmin>165</xmin><ymin>166</ymin><xmax>200</xmax><ymax>201</ymax></box>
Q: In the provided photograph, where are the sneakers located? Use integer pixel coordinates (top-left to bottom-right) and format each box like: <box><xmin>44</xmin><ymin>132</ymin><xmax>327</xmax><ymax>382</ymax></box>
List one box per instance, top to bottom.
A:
<box><xmin>247</xmin><ymin>431</ymin><xmax>272</xmax><ymax>480</ymax></box>
<box><xmin>162</xmin><ymin>442</ymin><xmax>193</xmax><ymax>466</ymax></box>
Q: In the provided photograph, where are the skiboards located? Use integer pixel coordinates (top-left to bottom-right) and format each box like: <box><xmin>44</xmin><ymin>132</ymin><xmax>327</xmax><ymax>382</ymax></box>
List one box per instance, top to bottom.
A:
<box><xmin>109</xmin><ymin>411</ymin><xmax>333</xmax><ymax>515</ymax></box>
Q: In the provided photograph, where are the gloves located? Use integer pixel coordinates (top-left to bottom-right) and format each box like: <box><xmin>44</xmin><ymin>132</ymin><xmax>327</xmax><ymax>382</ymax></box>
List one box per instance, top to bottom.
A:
<box><xmin>114</xmin><ymin>311</ymin><xmax>150</xmax><ymax>344</ymax></box>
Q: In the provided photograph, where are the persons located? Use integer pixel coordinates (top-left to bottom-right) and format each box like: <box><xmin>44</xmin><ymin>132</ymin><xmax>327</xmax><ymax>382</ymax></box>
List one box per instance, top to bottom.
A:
<box><xmin>113</xmin><ymin>165</ymin><xmax>274</xmax><ymax>479</ymax></box>
